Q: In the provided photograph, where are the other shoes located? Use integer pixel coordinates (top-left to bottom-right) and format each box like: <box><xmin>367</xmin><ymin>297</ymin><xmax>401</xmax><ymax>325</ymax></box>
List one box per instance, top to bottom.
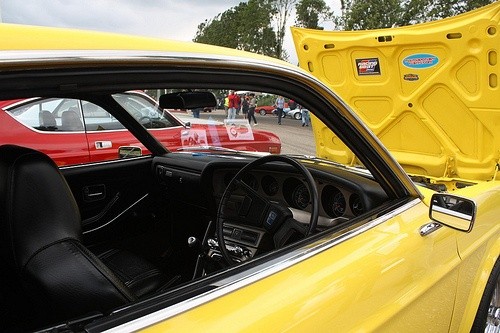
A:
<box><xmin>253</xmin><ymin>123</ymin><xmax>258</xmax><ymax>126</ymax></box>
<box><xmin>279</xmin><ymin>123</ymin><xmax>281</xmax><ymax>125</ymax></box>
<box><xmin>302</xmin><ymin>123</ymin><xmax>305</xmax><ymax>127</ymax></box>
<box><xmin>306</xmin><ymin>124</ymin><xmax>308</xmax><ymax>126</ymax></box>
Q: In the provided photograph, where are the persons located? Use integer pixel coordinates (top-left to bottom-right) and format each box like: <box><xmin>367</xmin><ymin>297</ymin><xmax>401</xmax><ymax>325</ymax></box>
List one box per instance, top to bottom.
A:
<box><xmin>227</xmin><ymin>90</ymin><xmax>249</xmax><ymax>120</ymax></box>
<box><xmin>246</xmin><ymin>93</ymin><xmax>258</xmax><ymax>126</ymax></box>
<box><xmin>275</xmin><ymin>95</ymin><xmax>284</xmax><ymax>125</ymax></box>
<box><xmin>288</xmin><ymin>99</ymin><xmax>297</xmax><ymax>110</ymax></box>
<box><xmin>302</xmin><ymin>106</ymin><xmax>309</xmax><ymax>127</ymax></box>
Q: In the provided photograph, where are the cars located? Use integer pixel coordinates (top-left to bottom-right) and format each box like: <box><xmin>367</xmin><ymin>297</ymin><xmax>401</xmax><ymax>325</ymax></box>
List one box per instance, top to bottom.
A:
<box><xmin>0</xmin><ymin>0</ymin><xmax>500</xmax><ymax>333</ymax></box>
<box><xmin>0</xmin><ymin>94</ymin><xmax>282</xmax><ymax>171</ymax></box>
<box><xmin>254</xmin><ymin>100</ymin><xmax>310</xmax><ymax>121</ymax></box>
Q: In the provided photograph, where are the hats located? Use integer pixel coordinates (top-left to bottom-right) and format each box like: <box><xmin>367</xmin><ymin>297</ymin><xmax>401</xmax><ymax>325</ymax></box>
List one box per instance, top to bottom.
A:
<box><xmin>249</xmin><ymin>94</ymin><xmax>254</xmax><ymax>96</ymax></box>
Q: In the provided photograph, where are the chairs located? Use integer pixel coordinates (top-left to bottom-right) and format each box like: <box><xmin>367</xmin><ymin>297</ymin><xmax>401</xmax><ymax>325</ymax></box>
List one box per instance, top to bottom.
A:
<box><xmin>69</xmin><ymin>107</ymin><xmax>81</xmax><ymax>117</ymax></box>
<box><xmin>39</xmin><ymin>111</ymin><xmax>56</xmax><ymax>125</ymax></box>
<box><xmin>62</xmin><ymin>111</ymin><xmax>82</xmax><ymax>131</ymax></box>
<box><xmin>0</xmin><ymin>145</ymin><xmax>130</xmax><ymax>333</ymax></box>
